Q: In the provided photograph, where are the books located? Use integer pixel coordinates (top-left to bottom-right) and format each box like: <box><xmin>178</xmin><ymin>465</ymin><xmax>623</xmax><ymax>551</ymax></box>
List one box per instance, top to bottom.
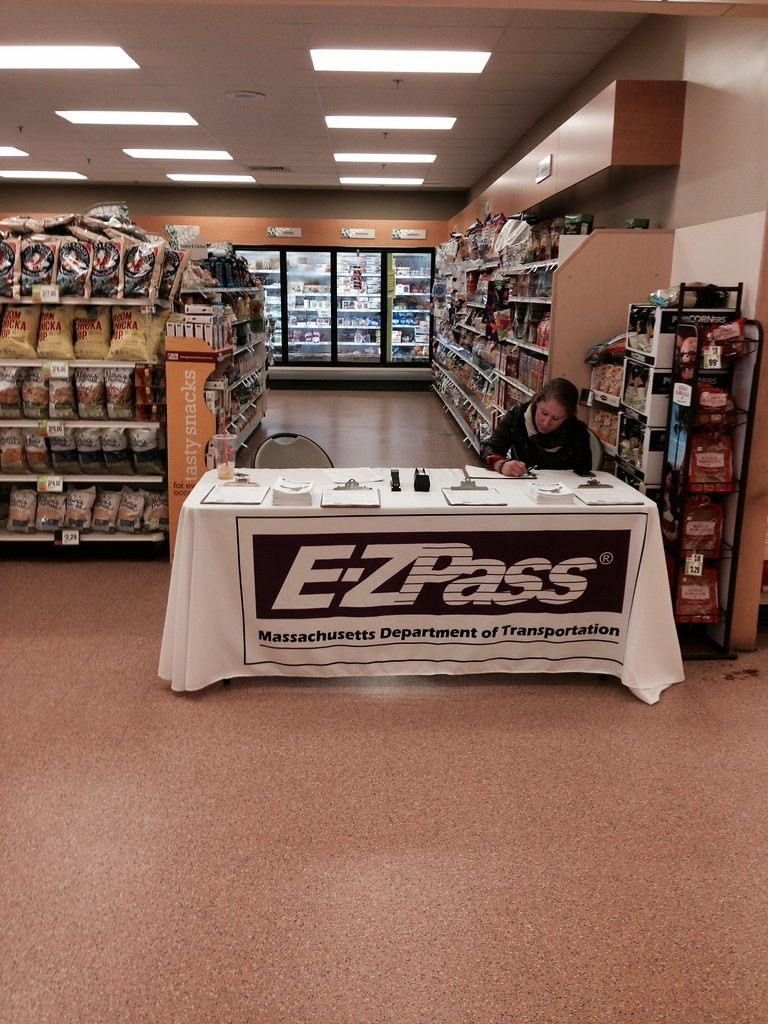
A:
<box><xmin>272</xmin><ymin>475</ymin><xmax>314</xmax><ymax>506</ymax></box>
<box><xmin>525</xmin><ymin>482</ymin><xmax>574</xmax><ymax>504</ymax></box>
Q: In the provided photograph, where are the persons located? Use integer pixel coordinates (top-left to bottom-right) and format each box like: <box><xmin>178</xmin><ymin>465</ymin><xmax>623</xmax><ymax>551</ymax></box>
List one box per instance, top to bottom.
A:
<box><xmin>479</xmin><ymin>378</ymin><xmax>592</xmax><ymax>477</ymax></box>
<box><xmin>660</xmin><ymin>471</ymin><xmax>677</xmax><ymax>516</ymax></box>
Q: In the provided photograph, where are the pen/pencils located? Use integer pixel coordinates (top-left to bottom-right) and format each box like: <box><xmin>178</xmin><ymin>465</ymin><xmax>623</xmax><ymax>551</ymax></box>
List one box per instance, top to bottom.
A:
<box><xmin>512</xmin><ymin>445</ymin><xmax>528</xmax><ymax>477</ymax></box>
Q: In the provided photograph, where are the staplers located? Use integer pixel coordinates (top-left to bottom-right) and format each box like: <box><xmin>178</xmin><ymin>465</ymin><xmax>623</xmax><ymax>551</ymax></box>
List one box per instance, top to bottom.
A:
<box><xmin>389</xmin><ymin>469</ymin><xmax>401</xmax><ymax>492</ymax></box>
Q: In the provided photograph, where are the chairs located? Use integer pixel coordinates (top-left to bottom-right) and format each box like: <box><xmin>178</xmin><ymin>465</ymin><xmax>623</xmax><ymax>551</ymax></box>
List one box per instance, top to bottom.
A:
<box><xmin>586</xmin><ymin>426</ymin><xmax>603</xmax><ymax>471</ymax></box>
<box><xmin>251</xmin><ymin>432</ymin><xmax>335</xmax><ymax>468</ymax></box>
<box><xmin>158</xmin><ymin>468</ymin><xmax>687</xmax><ymax>706</ymax></box>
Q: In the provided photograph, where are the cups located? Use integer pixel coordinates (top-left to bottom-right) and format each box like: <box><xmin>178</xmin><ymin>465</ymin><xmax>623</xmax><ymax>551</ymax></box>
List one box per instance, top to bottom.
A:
<box><xmin>212</xmin><ymin>435</ymin><xmax>238</xmax><ymax>479</ymax></box>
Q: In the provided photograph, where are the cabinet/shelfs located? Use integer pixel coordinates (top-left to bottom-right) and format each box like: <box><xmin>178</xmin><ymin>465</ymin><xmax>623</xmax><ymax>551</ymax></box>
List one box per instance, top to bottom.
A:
<box><xmin>491</xmin><ymin>234</ymin><xmax>592</xmax><ymax>438</ymax></box>
<box><xmin>232</xmin><ymin>245</ymin><xmax>285</xmax><ymax>366</ymax></box>
<box><xmin>284</xmin><ymin>245</ymin><xmax>334</xmax><ymax>367</ymax></box>
<box><xmin>334</xmin><ymin>246</ymin><xmax>386</xmax><ymax>367</ymax></box>
<box><xmin>386</xmin><ymin>246</ymin><xmax>435</xmax><ymax>367</ymax></box>
<box><xmin>430</xmin><ymin>259</ymin><xmax>505</xmax><ymax>456</ymax></box>
<box><xmin>657</xmin><ymin>281</ymin><xmax>764</xmax><ymax>661</ymax></box>
<box><xmin>0</xmin><ymin>296</ymin><xmax>175</xmax><ymax>542</ymax></box>
<box><xmin>587</xmin><ymin>388</ymin><xmax>621</xmax><ymax>459</ymax></box>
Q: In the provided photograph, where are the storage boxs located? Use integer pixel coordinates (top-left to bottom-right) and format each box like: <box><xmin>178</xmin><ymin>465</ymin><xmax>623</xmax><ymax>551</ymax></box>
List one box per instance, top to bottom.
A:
<box><xmin>562</xmin><ymin>213</ymin><xmax>593</xmax><ymax>235</ymax></box>
<box><xmin>626</xmin><ymin>219</ymin><xmax>649</xmax><ymax>229</ymax></box>
<box><xmin>165</xmin><ymin>286</ymin><xmax>268</xmax><ymax>565</ymax></box>
<box><xmin>614</xmin><ymin>302</ymin><xmax>736</xmax><ymax>505</ymax></box>
<box><xmin>166</xmin><ymin>303</ymin><xmax>227</xmax><ymax>470</ymax></box>
<box><xmin>493</xmin><ymin>344</ymin><xmax>548</xmax><ymax>412</ymax></box>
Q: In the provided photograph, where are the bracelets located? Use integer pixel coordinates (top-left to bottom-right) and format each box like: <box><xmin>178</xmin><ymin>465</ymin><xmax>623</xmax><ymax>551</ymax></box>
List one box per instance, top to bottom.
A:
<box><xmin>498</xmin><ymin>460</ymin><xmax>507</xmax><ymax>474</ymax></box>
